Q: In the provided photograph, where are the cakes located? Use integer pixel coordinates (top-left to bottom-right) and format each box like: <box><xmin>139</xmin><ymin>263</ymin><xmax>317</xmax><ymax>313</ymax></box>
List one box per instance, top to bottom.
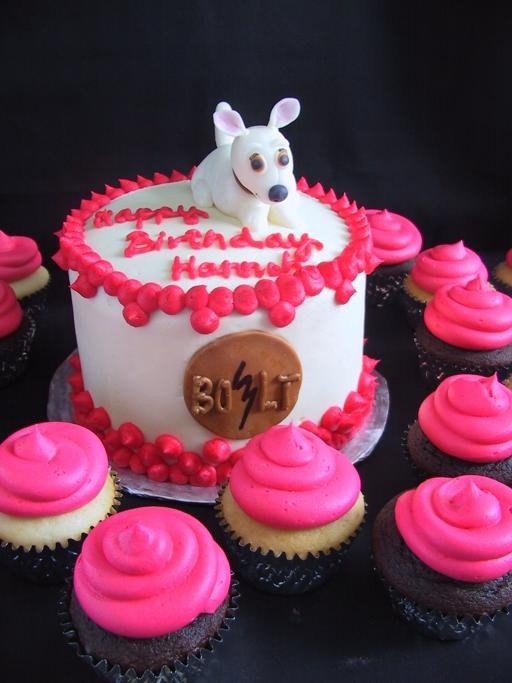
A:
<box><xmin>51</xmin><ymin>97</ymin><xmax>382</xmax><ymax>490</ymax></box>
<box><xmin>362</xmin><ymin>208</ymin><xmax>423</xmax><ymax>316</ymax></box>
<box><xmin>0</xmin><ymin>228</ymin><xmax>53</xmax><ymax>314</ymax></box>
<box><xmin>402</xmin><ymin>239</ymin><xmax>489</xmax><ymax>328</ymax></box>
<box><xmin>491</xmin><ymin>248</ymin><xmax>512</xmax><ymax>295</ymax></box>
<box><xmin>411</xmin><ymin>273</ymin><xmax>512</xmax><ymax>389</ymax></box>
<box><xmin>0</xmin><ymin>279</ymin><xmax>40</xmax><ymax>392</ymax></box>
<box><xmin>399</xmin><ymin>370</ymin><xmax>512</xmax><ymax>485</ymax></box>
<box><xmin>0</xmin><ymin>421</ymin><xmax>124</xmax><ymax>586</ymax></box>
<box><xmin>213</xmin><ymin>421</ymin><xmax>370</xmax><ymax>596</ymax></box>
<box><xmin>370</xmin><ymin>473</ymin><xmax>512</xmax><ymax>642</ymax></box>
<box><xmin>55</xmin><ymin>505</ymin><xmax>240</xmax><ymax>682</ymax></box>
